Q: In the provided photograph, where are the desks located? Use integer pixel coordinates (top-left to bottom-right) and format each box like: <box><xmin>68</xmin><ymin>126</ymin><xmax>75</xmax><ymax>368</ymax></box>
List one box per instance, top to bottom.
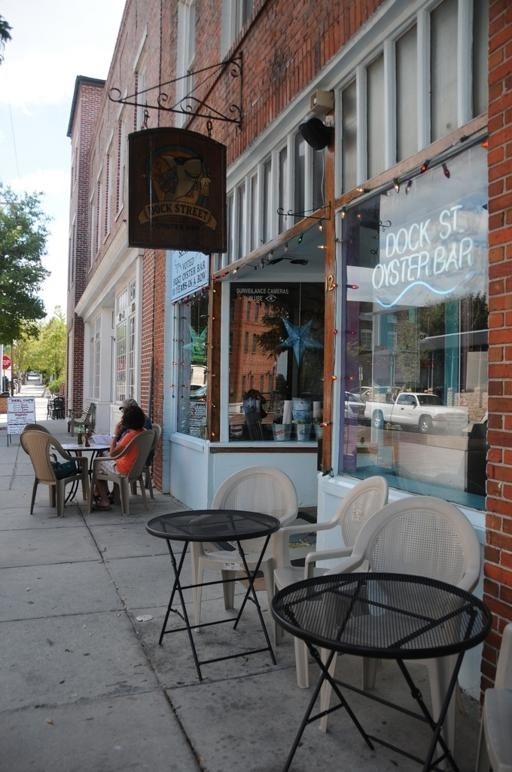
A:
<box><xmin>146</xmin><ymin>509</ymin><xmax>280</xmax><ymax>685</ymax></box>
<box><xmin>263</xmin><ymin>569</ymin><xmax>494</xmax><ymax>772</ymax></box>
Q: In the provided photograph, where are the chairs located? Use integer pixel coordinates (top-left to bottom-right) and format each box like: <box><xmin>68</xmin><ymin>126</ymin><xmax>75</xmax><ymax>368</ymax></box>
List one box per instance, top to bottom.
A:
<box><xmin>0</xmin><ymin>393</ymin><xmax>12</xmax><ymax>446</ymax></box>
<box><xmin>47</xmin><ymin>396</ymin><xmax>65</xmax><ymax>420</ymax></box>
<box><xmin>19</xmin><ymin>422</ymin><xmax>163</xmax><ymax>520</ymax></box>
<box><xmin>189</xmin><ymin>469</ymin><xmax>298</xmax><ymax>645</ymax></box>
<box><xmin>272</xmin><ymin>475</ymin><xmax>389</xmax><ymax>687</ymax></box>
<box><xmin>316</xmin><ymin>496</ymin><xmax>481</xmax><ymax>772</ymax></box>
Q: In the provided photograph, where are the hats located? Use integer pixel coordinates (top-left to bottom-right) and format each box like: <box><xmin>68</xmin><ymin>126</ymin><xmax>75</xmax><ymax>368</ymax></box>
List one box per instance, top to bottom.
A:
<box><xmin>118</xmin><ymin>398</ymin><xmax>138</xmax><ymax>411</ymax></box>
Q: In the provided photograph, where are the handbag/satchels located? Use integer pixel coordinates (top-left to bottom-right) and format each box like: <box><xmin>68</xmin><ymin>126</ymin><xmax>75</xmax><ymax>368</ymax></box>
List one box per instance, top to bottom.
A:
<box><xmin>49</xmin><ymin>454</ymin><xmax>77</xmax><ymax>480</ymax></box>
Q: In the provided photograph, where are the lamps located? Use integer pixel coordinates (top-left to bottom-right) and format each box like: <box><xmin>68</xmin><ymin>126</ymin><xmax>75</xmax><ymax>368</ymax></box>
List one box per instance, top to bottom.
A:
<box><xmin>298</xmin><ymin>117</ymin><xmax>335</xmax><ymax>151</ymax></box>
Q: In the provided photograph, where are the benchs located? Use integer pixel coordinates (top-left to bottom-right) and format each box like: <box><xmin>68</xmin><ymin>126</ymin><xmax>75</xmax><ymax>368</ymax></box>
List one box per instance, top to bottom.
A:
<box><xmin>67</xmin><ymin>402</ymin><xmax>95</xmax><ymax>437</ymax></box>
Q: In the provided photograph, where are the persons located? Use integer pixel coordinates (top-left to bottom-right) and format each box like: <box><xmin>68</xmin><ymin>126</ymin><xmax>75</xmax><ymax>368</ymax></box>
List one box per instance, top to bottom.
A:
<box><xmin>242</xmin><ymin>388</ymin><xmax>266</xmax><ymax>440</ymax></box>
<box><xmin>108</xmin><ymin>398</ymin><xmax>153</xmax><ymax>505</ymax></box>
<box><xmin>90</xmin><ymin>405</ymin><xmax>148</xmax><ymax>515</ymax></box>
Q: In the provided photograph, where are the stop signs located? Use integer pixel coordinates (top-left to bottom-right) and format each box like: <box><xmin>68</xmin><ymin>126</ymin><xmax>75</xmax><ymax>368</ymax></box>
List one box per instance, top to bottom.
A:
<box><xmin>2</xmin><ymin>356</ymin><xmax>11</xmax><ymax>369</ymax></box>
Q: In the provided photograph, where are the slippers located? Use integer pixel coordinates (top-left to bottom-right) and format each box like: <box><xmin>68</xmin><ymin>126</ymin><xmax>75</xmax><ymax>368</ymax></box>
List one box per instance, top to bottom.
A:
<box><xmin>92</xmin><ymin>502</ymin><xmax>112</xmax><ymax>512</ymax></box>
